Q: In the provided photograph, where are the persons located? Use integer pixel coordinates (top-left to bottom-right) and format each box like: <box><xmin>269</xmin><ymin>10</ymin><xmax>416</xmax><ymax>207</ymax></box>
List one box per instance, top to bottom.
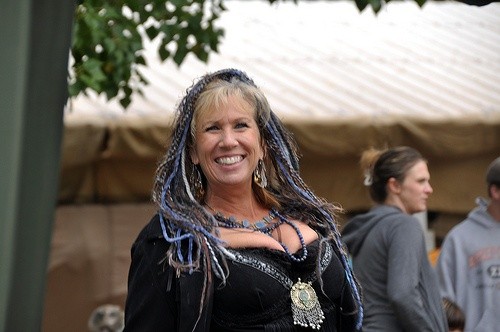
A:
<box><xmin>122</xmin><ymin>68</ymin><xmax>367</xmax><ymax>332</ymax></box>
<box><xmin>337</xmin><ymin>145</ymin><xmax>449</xmax><ymax>332</ymax></box>
<box><xmin>435</xmin><ymin>157</ymin><xmax>500</xmax><ymax>332</ymax></box>
<box><xmin>441</xmin><ymin>297</ymin><xmax>466</xmax><ymax>332</ymax></box>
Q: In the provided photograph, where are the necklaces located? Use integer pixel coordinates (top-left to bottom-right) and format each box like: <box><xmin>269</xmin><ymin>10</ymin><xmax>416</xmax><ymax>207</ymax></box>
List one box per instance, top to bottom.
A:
<box><xmin>202</xmin><ymin>199</ymin><xmax>279</xmax><ymax>230</ymax></box>
<box><xmin>218</xmin><ymin>206</ymin><xmax>307</xmax><ymax>262</ymax></box>
